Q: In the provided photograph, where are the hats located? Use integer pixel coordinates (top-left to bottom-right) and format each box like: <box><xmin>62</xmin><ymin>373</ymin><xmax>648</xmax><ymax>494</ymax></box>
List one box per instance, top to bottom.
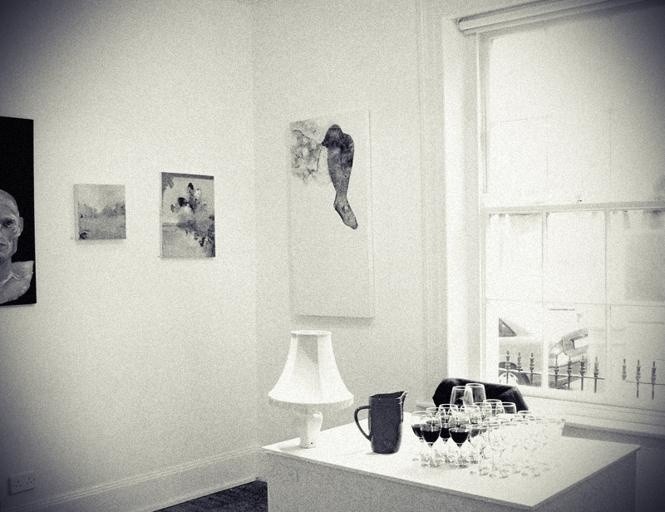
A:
<box><xmin>495</xmin><ymin>270</ymin><xmax>590</xmax><ymax>385</ymax></box>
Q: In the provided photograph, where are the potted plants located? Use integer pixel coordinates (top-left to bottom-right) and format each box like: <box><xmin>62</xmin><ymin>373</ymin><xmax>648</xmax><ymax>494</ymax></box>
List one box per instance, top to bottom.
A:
<box><xmin>73</xmin><ymin>184</ymin><xmax>127</xmax><ymax>244</ymax></box>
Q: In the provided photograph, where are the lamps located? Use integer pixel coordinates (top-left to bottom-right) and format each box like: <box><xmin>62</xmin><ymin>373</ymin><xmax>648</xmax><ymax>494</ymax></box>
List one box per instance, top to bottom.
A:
<box><xmin>431</xmin><ymin>380</ymin><xmax>528</xmax><ymax>414</ymax></box>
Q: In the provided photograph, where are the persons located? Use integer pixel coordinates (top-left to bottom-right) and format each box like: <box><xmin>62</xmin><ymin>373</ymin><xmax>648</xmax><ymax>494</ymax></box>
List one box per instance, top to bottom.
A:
<box><xmin>0</xmin><ymin>190</ymin><xmax>35</xmax><ymax>305</ymax></box>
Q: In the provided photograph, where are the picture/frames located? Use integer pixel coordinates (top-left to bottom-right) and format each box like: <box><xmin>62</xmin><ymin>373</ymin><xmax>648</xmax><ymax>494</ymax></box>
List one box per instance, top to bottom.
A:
<box><xmin>263</xmin><ymin>410</ymin><xmax>645</xmax><ymax>512</ymax></box>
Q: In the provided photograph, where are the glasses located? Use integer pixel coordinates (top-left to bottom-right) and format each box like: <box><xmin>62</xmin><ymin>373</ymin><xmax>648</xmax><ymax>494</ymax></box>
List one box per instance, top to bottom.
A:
<box><xmin>269</xmin><ymin>329</ymin><xmax>354</xmax><ymax>450</ymax></box>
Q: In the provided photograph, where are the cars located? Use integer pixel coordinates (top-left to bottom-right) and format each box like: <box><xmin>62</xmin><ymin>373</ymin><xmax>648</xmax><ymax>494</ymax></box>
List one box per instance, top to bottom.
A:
<box><xmin>353</xmin><ymin>392</ymin><xmax>405</xmax><ymax>453</ymax></box>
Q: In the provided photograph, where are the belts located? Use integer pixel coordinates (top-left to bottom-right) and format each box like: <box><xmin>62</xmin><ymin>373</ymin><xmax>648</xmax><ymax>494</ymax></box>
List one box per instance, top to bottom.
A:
<box><xmin>409</xmin><ymin>383</ymin><xmax>565</xmax><ymax>484</ymax></box>
